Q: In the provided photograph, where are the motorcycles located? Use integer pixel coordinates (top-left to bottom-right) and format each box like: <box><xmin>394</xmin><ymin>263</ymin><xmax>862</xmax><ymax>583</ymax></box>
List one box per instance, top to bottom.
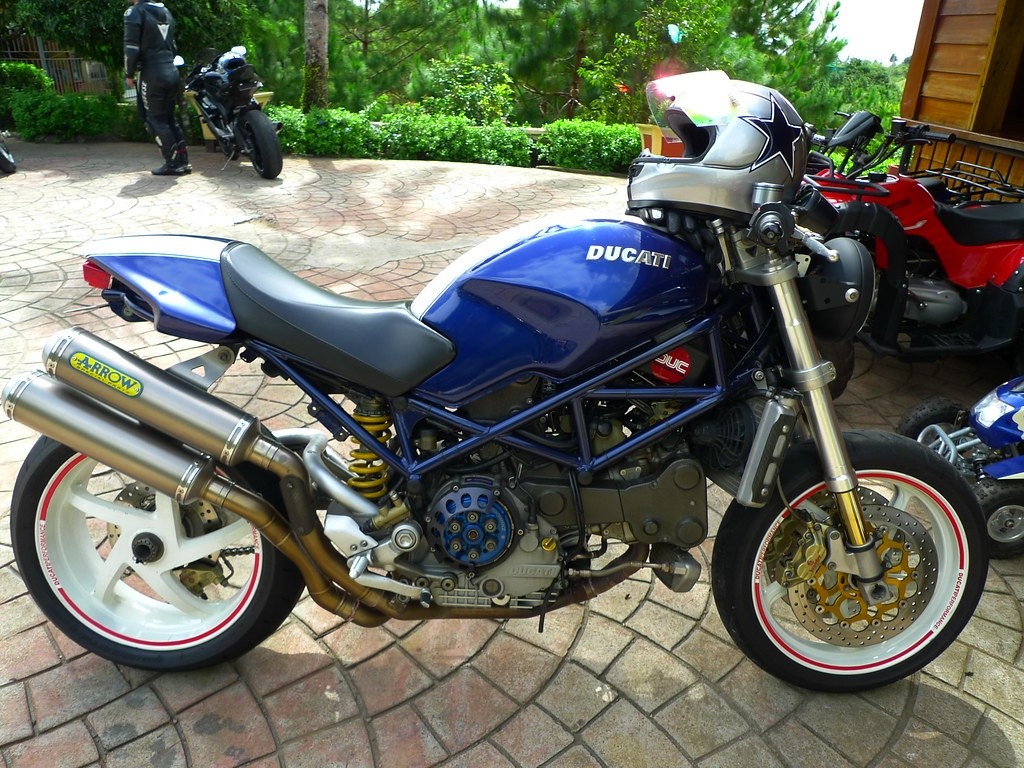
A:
<box><xmin>172</xmin><ymin>45</ymin><xmax>283</xmax><ymax>180</ymax></box>
<box><xmin>796</xmin><ymin>109</ymin><xmax>1024</xmax><ymax>406</ymax></box>
<box><xmin>0</xmin><ymin>129</ymin><xmax>17</xmax><ymax>174</ymax></box>
<box><xmin>1</xmin><ymin>179</ymin><xmax>992</xmax><ymax>676</ymax></box>
<box><xmin>895</xmin><ymin>375</ymin><xmax>1023</xmax><ymax>563</ymax></box>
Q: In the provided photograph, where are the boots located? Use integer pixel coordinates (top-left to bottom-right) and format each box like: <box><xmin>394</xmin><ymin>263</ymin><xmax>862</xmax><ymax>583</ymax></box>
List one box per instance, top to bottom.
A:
<box><xmin>172</xmin><ymin>124</ymin><xmax>193</xmax><ymax>174</ymax></box>
<box><xmin>151</xmin><ymin>133</ymin><xmax>185</xmax><ymax>175</ymax></box>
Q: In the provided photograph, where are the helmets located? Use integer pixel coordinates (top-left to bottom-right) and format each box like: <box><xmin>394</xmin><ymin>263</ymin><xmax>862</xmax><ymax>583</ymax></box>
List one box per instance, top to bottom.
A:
<box><xmin>216</xmin><ymin>51</ymin><xmax>247</xmax><ymax>84</ymax></box>
<box><xmin>627</xmin><ymin>71</ymin><xmax>808</xmax><ymax>223</ymax></box>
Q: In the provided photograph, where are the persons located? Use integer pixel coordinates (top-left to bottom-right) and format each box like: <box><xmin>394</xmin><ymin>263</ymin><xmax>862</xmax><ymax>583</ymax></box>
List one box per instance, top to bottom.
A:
<box><xmin>123</xmin><ymin>0</ymin><xmax>192</xmax><ymax>176</ymax></box>
